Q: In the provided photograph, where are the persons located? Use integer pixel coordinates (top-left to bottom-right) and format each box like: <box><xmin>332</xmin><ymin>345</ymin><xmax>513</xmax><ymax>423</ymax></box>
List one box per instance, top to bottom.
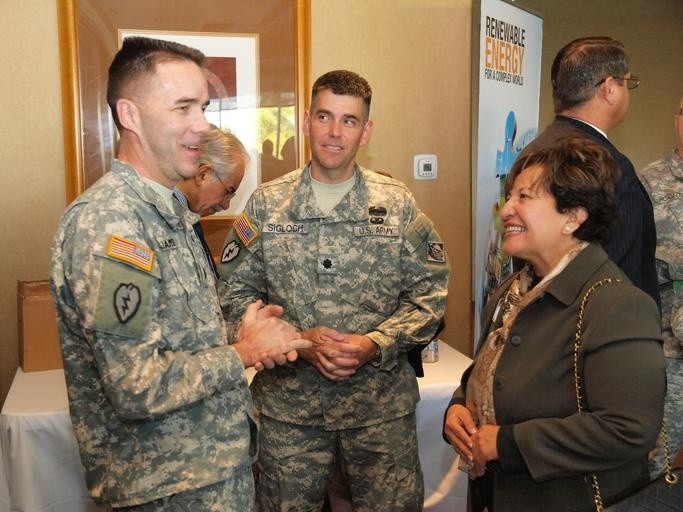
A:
<box><xmin>46</xmin><ymin>35</ymin><xmax>316</xmax><ymax>512</ymax></box>
<box><xmin>507</xmin><ymin>35</ymin><xmax>660</xmax><ymax>317</ymax></box>
<box><xmin>212</xmin><ymin>69</ymin><xmax>450</xmax><ymax>510</ymax></box>
<box><xmin>636</xmin><ymin>98</ymin><xmax>683</xmax><ymax>480</ymax></box>
<box><xmin>170</xmin><ymin>125</ymin><xmax>245</xmax><ymax>279</ymax></box>
<box><xmin>441</xmin><ymin>140</ymin><xmax>683</xmax><ymax>512</ymax></box>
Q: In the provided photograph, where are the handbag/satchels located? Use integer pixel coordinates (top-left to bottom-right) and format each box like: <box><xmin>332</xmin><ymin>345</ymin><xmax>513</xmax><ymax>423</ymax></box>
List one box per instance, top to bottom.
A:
<box><xmin>599</xmin><ymin>468</ymin><xmax>683</xmax><ymax>512</ymax></box>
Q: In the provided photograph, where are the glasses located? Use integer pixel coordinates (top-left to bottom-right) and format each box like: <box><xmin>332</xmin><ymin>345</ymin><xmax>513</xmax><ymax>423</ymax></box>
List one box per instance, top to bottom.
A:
<box><xmin>593</xmin><ymin>76</ymin><xmax>640</xmax><ymax>89</ymax></box>
<box><xmin>212</xmin><ymin>172</ymin><xmax>237</xmax><ymax>202</ymax></box>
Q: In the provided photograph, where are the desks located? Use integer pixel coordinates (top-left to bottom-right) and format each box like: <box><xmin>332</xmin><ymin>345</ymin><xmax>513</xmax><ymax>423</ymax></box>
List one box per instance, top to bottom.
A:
<box><xmin>3</xmin><ymin>340</ymin><xmax>484</xmax><ymax>510</ymax></box>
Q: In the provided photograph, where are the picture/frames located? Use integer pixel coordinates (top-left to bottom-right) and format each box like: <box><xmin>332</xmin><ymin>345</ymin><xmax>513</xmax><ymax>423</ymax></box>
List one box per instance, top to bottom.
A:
<box><xmin>55</xmin><ymin>0</ymin><xmax>325</xmax><ymax>245</ymax></box>
